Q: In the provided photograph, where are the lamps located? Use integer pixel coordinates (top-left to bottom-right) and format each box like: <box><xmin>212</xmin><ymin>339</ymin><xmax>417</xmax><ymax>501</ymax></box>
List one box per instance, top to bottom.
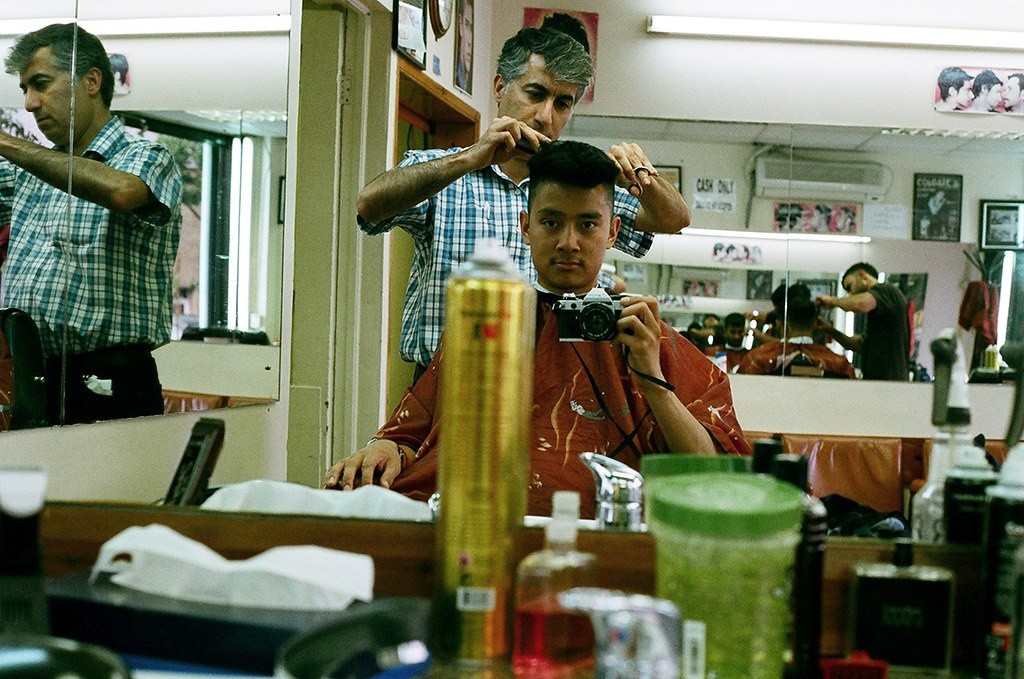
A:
<box><xmin>644</xmin><ymin>16</ymin><xmax>1023</xmax><ymax>53</ymax></box>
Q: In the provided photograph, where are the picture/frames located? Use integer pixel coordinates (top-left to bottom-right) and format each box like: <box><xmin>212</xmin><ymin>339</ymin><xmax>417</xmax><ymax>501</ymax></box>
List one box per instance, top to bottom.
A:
<box><xmin>391</xmin><ymin>0</ymin><xmax>427</xmax><ymax>69</ymax></box>
<box><xmin>453</xmin><ymin>0</ymin><xmax>474</xmax><ymax>98</ymax></box>
<box><xmin>652</xmin><ymin>165</ymin><xmax>683</xmax><ymax>195</ymax></box>
<box><xmin>911</xmin><ymin>173</ymin><xmax>963</xmax><ymax>243</ymax></box>
<box><xmin>979</xmin><ymin>199</ymin><xmax>1024</xmax><ymax>251</ymax></box>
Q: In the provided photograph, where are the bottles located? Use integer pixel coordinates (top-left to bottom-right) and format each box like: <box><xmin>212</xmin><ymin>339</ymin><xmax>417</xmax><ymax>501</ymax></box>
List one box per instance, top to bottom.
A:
<box><xmin>435</xmin><ymin>238</ymin><xmax>537</xmax><ymax>659</ymax></box>
<box><xmin>912</xmin><ymin>405</ymin><xmax>973</xmax><ymax>541</ymax></box>
<box><xmin>751</xmin><ymin>438</ymin><xmax>786</xmax><ymax>476</ymax></box>
<box><xmin>978</xmin><ymin>441</ymin><xmax>1024</xmax><ymax>679</ymax></box>
<box><xmin>943</xmin><ymin>446</ymin><xmax>998</xmax><ymax>542</ymax></box>
<box><xmin>772</xmin><ymin>454</ymin><xmax>827</xmax><ymax>679</ymax></box>
<box><xmin>641</xmin><ymin>455</ymin><xmax>752</xmax><ymax>529</ymax></box>
<box><xmin>648</xmin><ymin>473</ymin><xmax>807</xmax><ymax>679</ymax></box>
<box><xmin>513</xmin><ymin>519</ymin><xmax>605</xmax><ymax>679</ymax></box>
<box><xmin>844</xmin><ymin>542</ymin><xmax>954</xmax><ymax>668</ymax></box>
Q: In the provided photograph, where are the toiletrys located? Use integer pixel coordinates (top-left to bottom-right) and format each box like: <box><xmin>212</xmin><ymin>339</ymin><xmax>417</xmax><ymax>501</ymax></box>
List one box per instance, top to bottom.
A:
<box><xmin>908</xmin><ymin>325</ymin><xmax>999</xmax><ymax>540</ymax></box>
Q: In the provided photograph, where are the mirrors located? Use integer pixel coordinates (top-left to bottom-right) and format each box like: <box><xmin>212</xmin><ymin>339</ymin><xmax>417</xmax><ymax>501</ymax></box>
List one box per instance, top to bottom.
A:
<box><xmin>0</xmin><ymin>1</ymin><xmax>1023</xmax><ymax>522</ymax></box>
<box><xmin>108</xmin><ymin>110</ymin><xmax>288</xmax><ymax>345</ymax></box>
<box><xmin>615</xmin><ymin>262</ymin><xmax>840</xmax><ymax>373</ymax></box>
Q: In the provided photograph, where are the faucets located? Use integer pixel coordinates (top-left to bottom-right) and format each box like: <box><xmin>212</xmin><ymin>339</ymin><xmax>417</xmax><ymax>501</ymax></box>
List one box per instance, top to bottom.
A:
<box><xmin>578</xmin><ymin>449</ymin><xmax>645</xmax><ymax>532</ymax></box>
<box><xmin>556</xmin><ymin>584</ymin><xmax>684</xmax><ymax>678</ymax></box>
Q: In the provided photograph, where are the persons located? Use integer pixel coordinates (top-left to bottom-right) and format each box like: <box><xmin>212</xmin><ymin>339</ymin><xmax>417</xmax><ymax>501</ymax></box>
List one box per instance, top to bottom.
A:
<box><xmin>0</xmin><ymin>23</ymin><xmax>183</xmax><ymax>430</ymax></box>
<box><xmin>357</xmin><ymin>25</ymin><xmax>691</xmax><ymax>390</ymax></box>
<box><xmin>934</xmin><ymin>65</ymin><xmax>1024</xmax><ymax>116</ymax></box>
<box><xmin>324</xmin><ymin>140</ymin><xmax>751</xmax><ymax>530</ymax></box>
<box><xmin>775</xmin><ymin>201</ymin><xmax>858</xmax><ymax>236</ymax></box>
<box><xmin>672</xmin><ymin>244</ymin><xmax>912</xmax><ymax>382</ymax></box>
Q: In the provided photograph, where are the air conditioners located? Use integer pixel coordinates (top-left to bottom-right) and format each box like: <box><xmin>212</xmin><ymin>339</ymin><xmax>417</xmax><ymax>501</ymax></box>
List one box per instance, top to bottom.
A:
<box><xmin>755</xmin><ymin>156</ymin><xmax>887</xmax><ymax>203</ymax></box>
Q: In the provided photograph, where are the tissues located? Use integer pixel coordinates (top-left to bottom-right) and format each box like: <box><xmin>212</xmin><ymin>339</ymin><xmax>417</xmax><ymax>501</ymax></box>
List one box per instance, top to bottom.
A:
<box><xmin>200</xmin><ymin>475</ymin><xmax>435</xmax><ymax>524</ymax></box>
<box><xmin>45</xmin><ymin>520</ymin><xmax>378</xmax><ymax>675</ymax></box>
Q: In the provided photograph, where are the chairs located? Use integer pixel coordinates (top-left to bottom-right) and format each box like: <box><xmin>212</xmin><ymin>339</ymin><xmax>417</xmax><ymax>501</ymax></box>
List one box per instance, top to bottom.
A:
<box><xmin>741</xmin><ymin>432</ymin><xmax>1009</xmax><ymax>514</ymax></box>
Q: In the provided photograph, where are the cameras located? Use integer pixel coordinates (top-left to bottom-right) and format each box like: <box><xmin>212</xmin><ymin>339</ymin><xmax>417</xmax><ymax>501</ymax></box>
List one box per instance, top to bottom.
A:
<box><xmin>555</xmin><ymin>288</ymin><xmax>641</xmax><ymax>343</ymax></box>
